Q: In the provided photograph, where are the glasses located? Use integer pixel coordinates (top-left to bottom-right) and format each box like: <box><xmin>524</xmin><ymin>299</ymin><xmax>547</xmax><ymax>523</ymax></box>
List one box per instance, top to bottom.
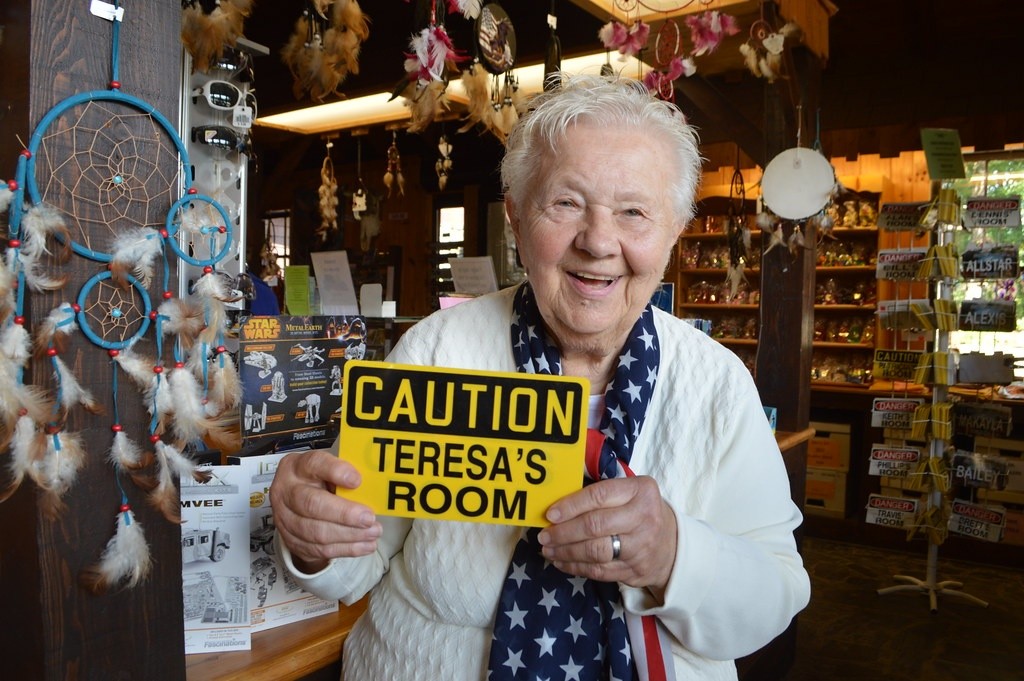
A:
<box><xmin>210</xmin><ymin>45</ymin><xmax>258</xmax><ymax>80</ymax></box>
<box><xmin>193</xmin><ymin>80</ymin><xmax>257</xmax><ymax>122</ymax></box>
<box><xmin>194</xmin><ymin>125</ymin><xmax>253</xmax><ymax>156</ymax></box>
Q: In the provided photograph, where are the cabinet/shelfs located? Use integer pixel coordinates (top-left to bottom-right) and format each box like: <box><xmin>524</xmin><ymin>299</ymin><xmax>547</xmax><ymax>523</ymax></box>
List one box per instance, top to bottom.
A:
<box><xmin>674</xmin><ymin>224</ymin><xmax>879</xmax><ymax>396</ymax></box>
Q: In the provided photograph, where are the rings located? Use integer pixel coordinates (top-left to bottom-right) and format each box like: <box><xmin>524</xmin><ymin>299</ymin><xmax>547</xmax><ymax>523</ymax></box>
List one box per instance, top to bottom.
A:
<box><xmin>611</xmin><ymin>535</ymin><xmax>622</xmax><ymax>563</ymax></box>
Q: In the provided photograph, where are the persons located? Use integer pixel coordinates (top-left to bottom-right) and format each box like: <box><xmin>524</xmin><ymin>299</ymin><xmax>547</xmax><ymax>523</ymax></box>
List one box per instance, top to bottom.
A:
<box><xmin>269</xmin><ymin>72</ymin><xmax>811</xmax><ymax>681</ymax></box>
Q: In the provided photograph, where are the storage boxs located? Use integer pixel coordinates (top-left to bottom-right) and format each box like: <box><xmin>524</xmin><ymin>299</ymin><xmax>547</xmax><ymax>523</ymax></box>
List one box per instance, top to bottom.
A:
<box><xmin>801</xmin><ymin>422</ymin><xmax>853</xmax><ymax>521</ymax></box>
<box><xmin>878</xmin><ymin>429</ymin><xmax>1024</xmax><ymax>552</ymax></box>
